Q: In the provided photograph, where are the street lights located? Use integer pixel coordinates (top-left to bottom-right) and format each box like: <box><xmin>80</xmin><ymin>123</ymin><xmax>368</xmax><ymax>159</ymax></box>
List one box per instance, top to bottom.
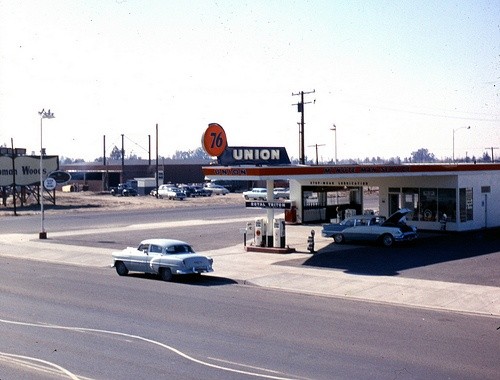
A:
<box><xmin>37</xmin><ymin>111</ymin><xmax>55</xmax><ymax>233</ymax></box>
<box><xmin>453</xmin><ymin>125</ymin><xmax>470</xmax><ymax>162</ymax></box>
<box><xmin>330</xmin><ymin>128</ymin><xmax>338</xmax><ymax>165</ymax></box>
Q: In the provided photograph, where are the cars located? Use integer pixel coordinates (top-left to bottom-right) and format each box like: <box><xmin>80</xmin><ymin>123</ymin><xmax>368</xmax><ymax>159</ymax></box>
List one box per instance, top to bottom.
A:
<box><xmin>151</xmin><ymin>183</ymin><xmax>229</xmax><ymax>200</ymax></box>
<box><xmin>242</xmin><ymin>188</ymin><xmax>289</xmax><ymax>200</ymax></box>
<box><xmin>321</xmin><ymin>209</ymin><xmax>418</xmax><ymax>246</ymax></box>
<box><xmin>110</xmin><ymin>239</ymin><xmax>214</xmax><ymax>282</ymax></box>
